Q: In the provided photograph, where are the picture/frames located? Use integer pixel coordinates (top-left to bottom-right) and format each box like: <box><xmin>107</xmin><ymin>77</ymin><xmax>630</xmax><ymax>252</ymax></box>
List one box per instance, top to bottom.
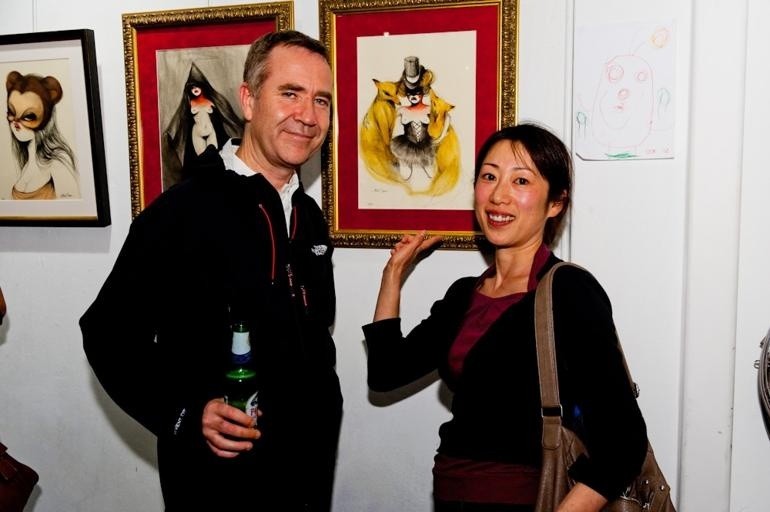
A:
<box><xmin>122</xmin><ymin>0</ymin><xmax>297</xmax><ymax>231</ymax></box>
<box><xmin>316</xmin><ymin>0</ymin><xmax>518</xmax><ymax>253</ymax></box>
<box><xmin>1</xmin><ymin>28</ymin><xmax>112</xmax><ymax>229</ymax></box>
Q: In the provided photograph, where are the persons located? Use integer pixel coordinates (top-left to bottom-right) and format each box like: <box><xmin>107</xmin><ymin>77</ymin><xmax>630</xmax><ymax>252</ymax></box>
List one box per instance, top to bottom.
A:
<box><xmin>82</xmin><ymin>29</ymin><xmax>344</xmax><ymax>512</ymax></box>
<box><xmin>362</xmin><ymin>121</ymin><xmax>648</xmax><ymax>511</ymax></box>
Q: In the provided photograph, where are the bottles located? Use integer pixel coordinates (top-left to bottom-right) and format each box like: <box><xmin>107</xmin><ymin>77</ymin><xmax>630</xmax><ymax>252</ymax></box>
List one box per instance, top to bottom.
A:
<box><xmin>222</xmin><ymin>315</ymin><xmax>260</xmax><ymax>444</ymax></box>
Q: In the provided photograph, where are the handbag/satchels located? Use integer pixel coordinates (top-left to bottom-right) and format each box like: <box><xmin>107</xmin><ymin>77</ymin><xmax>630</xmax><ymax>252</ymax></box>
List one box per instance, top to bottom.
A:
<box><xmin>534</xmin><ymin>261</ymin><xmax>676</xmax><ymax>511</ymax></box>
